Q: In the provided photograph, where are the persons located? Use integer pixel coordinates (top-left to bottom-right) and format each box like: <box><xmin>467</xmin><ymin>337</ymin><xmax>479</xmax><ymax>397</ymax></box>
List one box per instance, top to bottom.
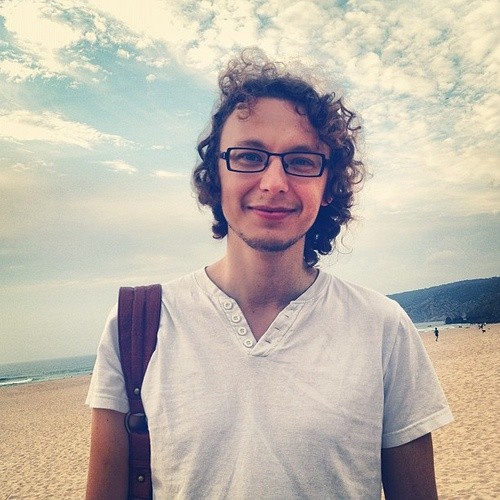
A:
<box><xmin>83</xmin><ymin>48</ymin><xmax>460</xmax><ymax>500</ymax></box>
<box><xmin>433</xmin><ymin>327</ymin><xmax>439</xmax><ymax>341</ymax></box>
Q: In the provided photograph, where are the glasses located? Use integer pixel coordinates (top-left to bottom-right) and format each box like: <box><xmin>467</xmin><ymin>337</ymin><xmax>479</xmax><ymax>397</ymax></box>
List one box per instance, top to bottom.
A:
<box><xmin>215</xmin><ymin>146</ymin><xmax>330</xmax><ymax>178</ymax></box>
